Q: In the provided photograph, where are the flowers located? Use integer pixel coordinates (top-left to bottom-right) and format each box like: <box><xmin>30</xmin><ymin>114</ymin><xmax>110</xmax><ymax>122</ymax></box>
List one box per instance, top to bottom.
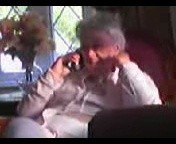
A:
<box><xmin>0</xmin><ymin>10</ymin><xmax>55</xmax><ymax>81</ymax></box>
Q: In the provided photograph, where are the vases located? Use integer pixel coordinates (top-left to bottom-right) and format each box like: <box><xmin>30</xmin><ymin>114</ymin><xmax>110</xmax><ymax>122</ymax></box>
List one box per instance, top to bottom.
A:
<box><xmin>17</xmin><ymin>81</ymin><xmax>36</xmax><ymax>95</ymax></box>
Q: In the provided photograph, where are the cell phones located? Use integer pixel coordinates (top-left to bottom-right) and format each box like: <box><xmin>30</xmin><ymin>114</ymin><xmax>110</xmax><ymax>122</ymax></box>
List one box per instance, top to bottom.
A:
<box><xmin>66</xmin><ymin>49</ymin><xmax>83</xmax><ymax>71</ymax></box>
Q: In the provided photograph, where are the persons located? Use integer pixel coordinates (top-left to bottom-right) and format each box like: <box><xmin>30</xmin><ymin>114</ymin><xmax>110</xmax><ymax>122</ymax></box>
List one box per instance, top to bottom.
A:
<box><xmin>2</xmin><ymin>11</ymin><xmax>162</xmax><ymax>138</ymax></box>
